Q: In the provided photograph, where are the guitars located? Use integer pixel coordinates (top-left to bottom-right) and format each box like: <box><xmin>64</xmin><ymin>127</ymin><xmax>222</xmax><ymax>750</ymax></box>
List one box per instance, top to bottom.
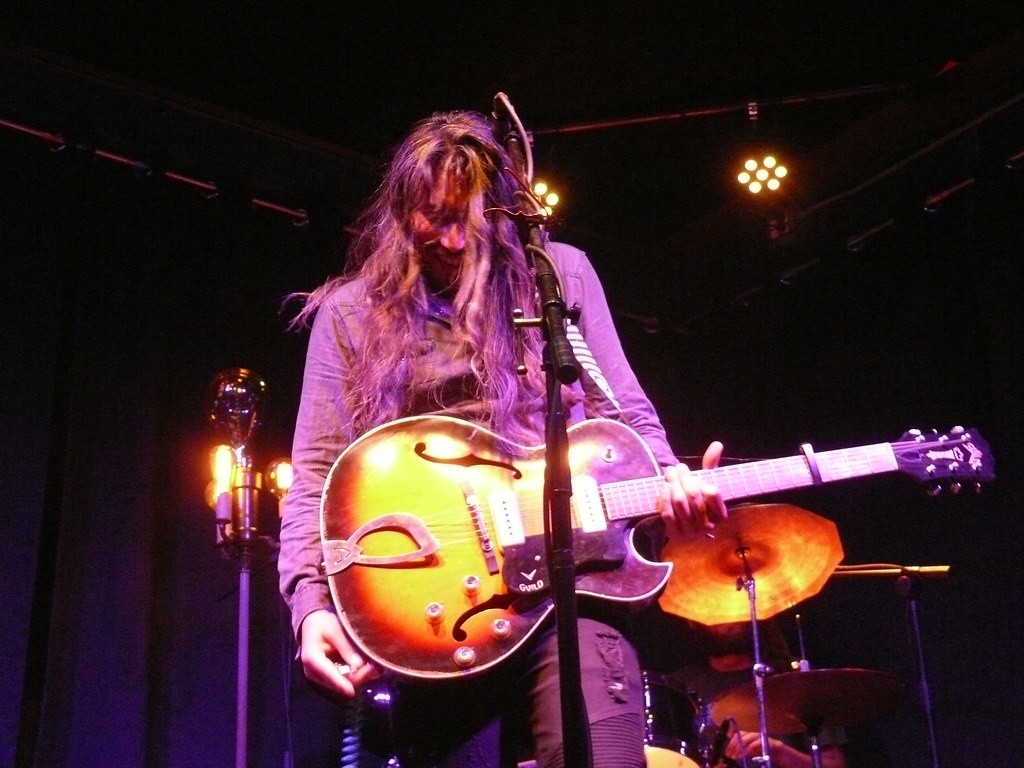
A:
<box><xmin>321</xmin><ymin>420</ymin><xmax>995</xmax><ymax>688</ymax></box>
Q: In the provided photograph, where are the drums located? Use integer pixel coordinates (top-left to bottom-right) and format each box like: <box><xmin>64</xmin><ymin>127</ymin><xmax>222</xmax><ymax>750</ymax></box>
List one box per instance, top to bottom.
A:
<box><xmin>639</xmin><ymin>729</ymin><xmax>712</xmax><ymax>768</ymax></box>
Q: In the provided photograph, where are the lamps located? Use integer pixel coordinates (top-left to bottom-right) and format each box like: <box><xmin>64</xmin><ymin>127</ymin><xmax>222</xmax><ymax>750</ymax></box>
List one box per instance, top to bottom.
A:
<box><xmin>205</xmin><ymin>369</ymin><xmax>292</xmax><ymax>768</ymax></box>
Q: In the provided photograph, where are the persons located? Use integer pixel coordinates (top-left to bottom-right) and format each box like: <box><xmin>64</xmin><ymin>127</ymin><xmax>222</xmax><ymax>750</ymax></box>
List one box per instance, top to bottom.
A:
<box><xmin>277</xmin><ymin>112</ymin><xmax>729</xmax><ymax>768</ymax></box>
<box><xmin>709</xmin><ymin>636</ymin><xmax>848</xmax><ymax>768</ymax></box>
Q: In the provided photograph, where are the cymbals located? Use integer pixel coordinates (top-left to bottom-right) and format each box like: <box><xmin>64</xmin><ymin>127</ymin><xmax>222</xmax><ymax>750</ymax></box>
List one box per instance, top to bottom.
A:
<box><xmin>656</xmin><ymin>502</ymin><xmax>845</xmax><ymax>626</ymax></box>
<box><xmin>710</xmin><ymin>667</ymin><xmax>901</xmax><ymax>742</ymax></box>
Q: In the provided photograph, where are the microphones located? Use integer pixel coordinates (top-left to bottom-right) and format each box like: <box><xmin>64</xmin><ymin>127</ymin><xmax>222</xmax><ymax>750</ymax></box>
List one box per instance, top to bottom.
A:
<box><xmin>489</xmin><ymin>92</ymin><xmax>513</xmax><ymax>145</ymax></box>
<box><xmin>202</xmin><ymin>368</ymin><xmax>268</xmax><ymax>560</ymax></box>
<box><xmin>711</xmin><ymin>719</ymin><xmax>732</xmax><ymax>766</ymax></box>
